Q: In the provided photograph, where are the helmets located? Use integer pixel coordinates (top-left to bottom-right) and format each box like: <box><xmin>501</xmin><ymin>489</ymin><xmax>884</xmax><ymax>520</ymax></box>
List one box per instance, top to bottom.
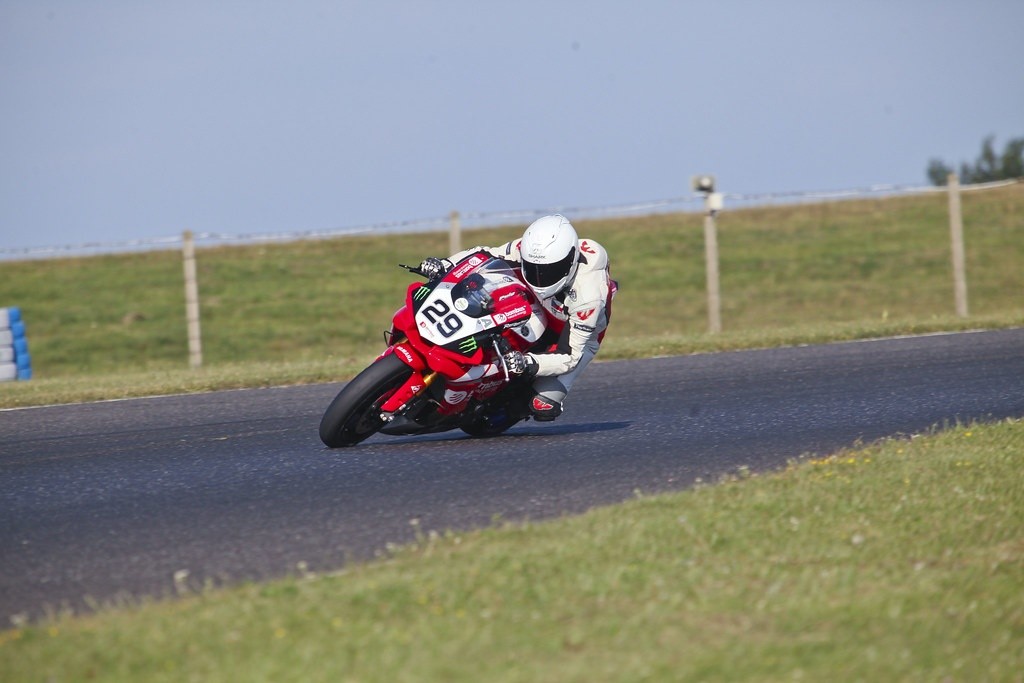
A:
<box><xmin>520</xmin><ymin>215</ymin><xmax>577</xmax><ymax>296</ymax></box>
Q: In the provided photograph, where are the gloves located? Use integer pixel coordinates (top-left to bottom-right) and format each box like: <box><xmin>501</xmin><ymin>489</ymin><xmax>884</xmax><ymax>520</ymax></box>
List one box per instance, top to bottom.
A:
<box><xmin>420</xmin><ymin>256</ymin><xmax>453</xmax><ymax>279</ymax></box>
<box><xmin>503</xmin><ymin>349</ymin><xmax>540</xmax><ymax>376</ymax></box>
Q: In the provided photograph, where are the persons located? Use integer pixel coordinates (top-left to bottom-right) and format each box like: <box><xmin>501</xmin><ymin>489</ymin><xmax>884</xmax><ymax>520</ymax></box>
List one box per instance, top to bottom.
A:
<box><xmin>419</xmin><ymin>213</ymin><xmax>617</xmax><ymax>421</ymax></box>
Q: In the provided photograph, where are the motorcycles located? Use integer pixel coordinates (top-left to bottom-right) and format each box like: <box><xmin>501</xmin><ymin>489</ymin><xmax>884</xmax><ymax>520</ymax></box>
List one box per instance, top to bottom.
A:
<box><xmin>320</xmin><ymin>251</ymin><xmax>618</xmax><ymax>447</ymax></box>
